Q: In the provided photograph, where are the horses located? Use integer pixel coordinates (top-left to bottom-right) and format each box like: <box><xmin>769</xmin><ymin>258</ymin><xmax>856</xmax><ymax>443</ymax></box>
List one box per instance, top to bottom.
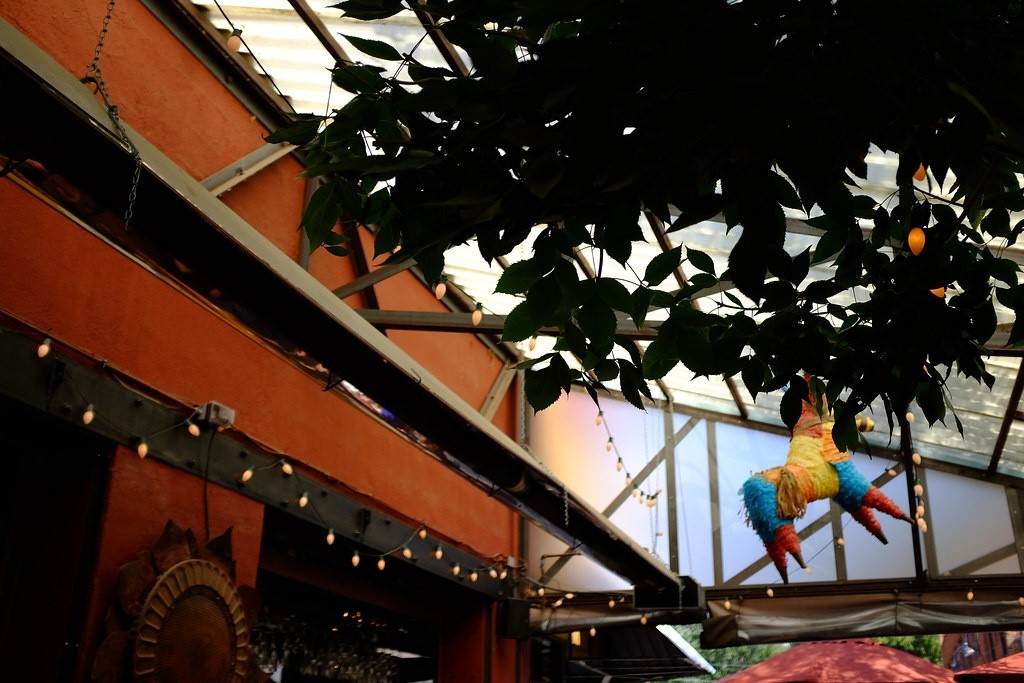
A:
<box><xmin>743</xmin><ymin>373</ymin><xmax>916</xmax><ymax>586</ymax></box>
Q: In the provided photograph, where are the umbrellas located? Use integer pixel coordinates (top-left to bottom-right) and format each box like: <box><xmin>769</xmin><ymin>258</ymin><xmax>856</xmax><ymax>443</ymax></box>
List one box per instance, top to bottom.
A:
<box><xmin>715</xmin><ymin>638</ymin><xmax>956</xmax><ymax>683</ymax></box>
<box><xmin>953</xmin><ymin>651</ymin><xmax>1024</xmax><ymax>683</ymax></box>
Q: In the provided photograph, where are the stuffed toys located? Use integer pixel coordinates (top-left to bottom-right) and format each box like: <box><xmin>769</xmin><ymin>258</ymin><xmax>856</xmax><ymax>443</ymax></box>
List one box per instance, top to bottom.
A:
<box><xmin>738</xmin><ymin>374</ymin><xmax>918</xmax><ymax>585</ymax></box>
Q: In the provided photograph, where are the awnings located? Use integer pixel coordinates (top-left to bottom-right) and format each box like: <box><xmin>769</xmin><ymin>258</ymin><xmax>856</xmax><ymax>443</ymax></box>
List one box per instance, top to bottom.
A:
<box><xmin>591</xmin><ymin>623</ymin><xmax>716</xmax><ymax>681</ymax></box>
